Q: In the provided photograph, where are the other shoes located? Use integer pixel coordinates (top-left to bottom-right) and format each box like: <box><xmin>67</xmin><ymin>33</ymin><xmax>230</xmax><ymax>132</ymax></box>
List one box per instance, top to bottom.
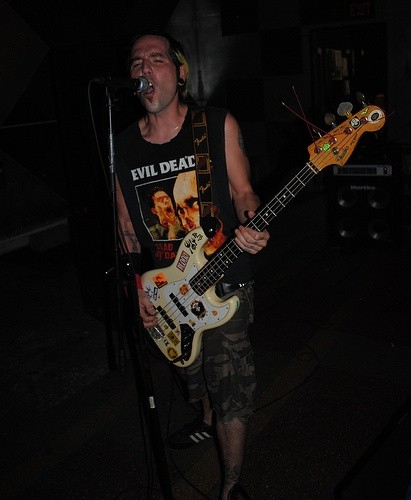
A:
<box><xmin>165</xmin><ymin>418</ymin><xmax>216</xmax><ymax>449</ymax></box>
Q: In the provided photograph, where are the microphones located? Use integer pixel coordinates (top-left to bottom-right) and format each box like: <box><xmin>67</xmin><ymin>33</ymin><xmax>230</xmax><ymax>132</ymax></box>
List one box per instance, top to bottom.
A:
<box><xmin>92</xmin><ymin>75</ymin><xmax>149</xmax><ymax>92</ymax></box>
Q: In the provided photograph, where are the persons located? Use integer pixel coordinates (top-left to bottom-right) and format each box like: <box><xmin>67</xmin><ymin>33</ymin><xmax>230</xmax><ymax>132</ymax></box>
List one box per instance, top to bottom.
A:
<box><xmin>114</xmin><ymin>28</ymin><xmax>270</xmax><ymax>499</ymax></box>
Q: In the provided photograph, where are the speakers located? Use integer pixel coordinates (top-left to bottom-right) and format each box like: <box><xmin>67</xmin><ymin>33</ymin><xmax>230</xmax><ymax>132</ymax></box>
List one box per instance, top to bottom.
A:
<box><xmin>323</xmin><ymin>159</ymin><xmax>403</xmax><ymax>255</ymax></box>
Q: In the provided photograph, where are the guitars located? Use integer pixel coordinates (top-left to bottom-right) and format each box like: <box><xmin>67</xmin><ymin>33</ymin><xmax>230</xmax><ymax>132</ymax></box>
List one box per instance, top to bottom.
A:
<box><xmin>138</xmin><ymin>90</ymin><xmax>387</xmax><ymax>368</ymax></box>
